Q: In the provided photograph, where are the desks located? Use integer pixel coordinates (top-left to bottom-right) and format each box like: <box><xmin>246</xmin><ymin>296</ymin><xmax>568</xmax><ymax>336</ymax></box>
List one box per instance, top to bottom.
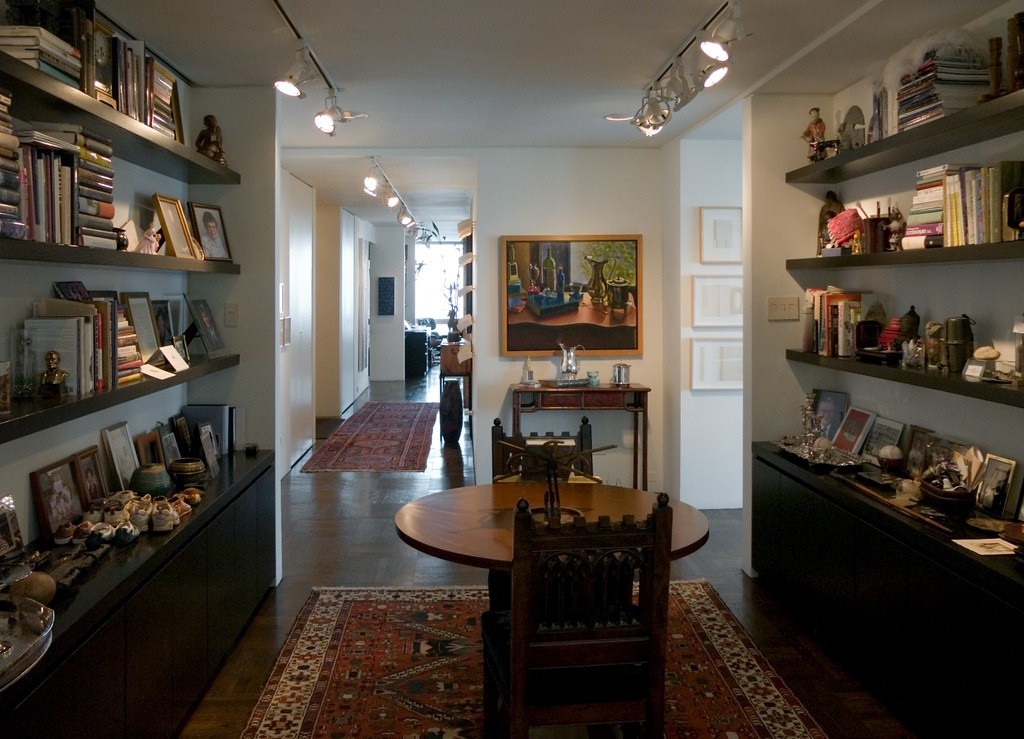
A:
<box><xmin>511</xmin><ymin>384</ymin><xmax>651</xmax><ymax>491</ymax></box>
<box><xmin>394</xmin><ymin>483</ymin><xmax>710</xmax><ymax>739</ymax></box>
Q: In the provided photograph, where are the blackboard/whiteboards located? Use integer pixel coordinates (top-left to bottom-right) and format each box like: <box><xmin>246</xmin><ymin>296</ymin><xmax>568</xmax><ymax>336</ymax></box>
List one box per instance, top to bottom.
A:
<box><xmin>378</xmin><ymin>277</ymin><xmax>394</xmax><ymax>316</ymax></box>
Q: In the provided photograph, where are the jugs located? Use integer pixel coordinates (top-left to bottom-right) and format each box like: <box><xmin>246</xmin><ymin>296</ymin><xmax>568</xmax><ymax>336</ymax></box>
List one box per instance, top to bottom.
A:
<box><xmin>559</xmin><ymin>343</ymin><xmax>585</xmax><ymax>379</ymax></box>
<box><xmin>613</xmin><ymin>363</ymin><xmax>632</xmax><ymax>386</ymax></box>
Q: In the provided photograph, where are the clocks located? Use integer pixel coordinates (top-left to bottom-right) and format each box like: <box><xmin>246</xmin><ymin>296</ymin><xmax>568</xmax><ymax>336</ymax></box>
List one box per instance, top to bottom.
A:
<box><xmin>94</xmin><ymin>16</ymin><xmax>117</xmax><ymax>110</ymax></box>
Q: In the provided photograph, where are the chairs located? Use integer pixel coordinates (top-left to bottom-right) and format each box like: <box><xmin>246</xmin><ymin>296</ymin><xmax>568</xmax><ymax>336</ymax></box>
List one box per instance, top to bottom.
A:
<box><xmin>417</xmin><ymin>317</ymin><xmax>449</xmax><ymax>358</ymax></box>
<box><xmin>486</xmin><ymin>417</ymin><xmax>673</xmax><ymax>739</ymax></box>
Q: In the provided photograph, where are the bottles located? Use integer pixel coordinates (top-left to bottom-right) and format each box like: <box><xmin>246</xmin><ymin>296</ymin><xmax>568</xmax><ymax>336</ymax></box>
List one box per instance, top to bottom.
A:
<box><xmin>523</xmin><ymin>354</ymin><xmax>533</xmax><ymax>383</ymax></box>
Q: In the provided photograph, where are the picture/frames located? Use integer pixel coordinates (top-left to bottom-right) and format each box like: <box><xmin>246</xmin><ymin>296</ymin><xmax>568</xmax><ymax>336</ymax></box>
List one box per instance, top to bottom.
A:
<box><xmin>172</xmin><ymin>79</ymin><xmax>185</xmax><ymax>144</ymax></box>
<box><xmin>0</xmin><ymin>194</ymin><xmax>235</xmax><ymax>566</ymax></box>
<box><xmin>690</xmin><ymin>206</ymin><xmax>743</xmax><ymax>391</ymax></box>
<box><xmin>500</xmin><ymin>234</ymin><xmax>645</xmax><ymax>358</ymax></box>
<box><xmin>279</xmin><ymin>282</ymin><xmax>291</xmax><ymax>347</ymax></box>
<box><xmin>812</xmin><ymin>389</ymin><xmax>1016</xmax><ymax>521</ymax></box>
<box><xmin>952</xmin><ymin>538</ymin><xmax>1018</xmax><ymax>557</ymax></box>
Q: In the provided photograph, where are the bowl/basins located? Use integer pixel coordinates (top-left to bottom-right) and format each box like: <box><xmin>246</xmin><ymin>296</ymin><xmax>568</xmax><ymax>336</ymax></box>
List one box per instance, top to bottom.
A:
<box><xmin>878</xmin><ymin>457</ymin><xmax>904</xmax><ymax>469</ymax></box>
<box><xmin>170</xmin><ymin>458</ymin><xmax>206</xmax><ymax>488</ymax></box>
<box><xmin>918</xmin><ymin>479</ymin><xmax>974</xmax><ymax>507</ymax></box>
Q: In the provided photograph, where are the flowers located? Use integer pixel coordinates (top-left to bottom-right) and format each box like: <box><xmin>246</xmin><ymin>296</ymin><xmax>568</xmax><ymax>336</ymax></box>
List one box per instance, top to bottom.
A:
<box><xmin>442</xmin><ymin>271</ymin><xmax>461</xmax><ymax>319</ymax></box>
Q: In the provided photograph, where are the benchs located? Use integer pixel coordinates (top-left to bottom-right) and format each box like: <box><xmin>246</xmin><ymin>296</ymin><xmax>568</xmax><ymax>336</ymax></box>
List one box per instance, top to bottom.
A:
<box><xmin>404</xmin><ymin>329</ymin><xmax>434</xmax><ymax>380</ymax></box>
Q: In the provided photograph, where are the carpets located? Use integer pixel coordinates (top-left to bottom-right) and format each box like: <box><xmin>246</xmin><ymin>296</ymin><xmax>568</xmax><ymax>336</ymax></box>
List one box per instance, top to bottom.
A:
<box><xmin>300</xmin><ymin>402</ymin><xmax>441</xmax><ymax>472</ymax></box>
<box><xmin>237</xmin><ymin>579</ymin><xmax>830</xmax><ymax>739</ymax></box>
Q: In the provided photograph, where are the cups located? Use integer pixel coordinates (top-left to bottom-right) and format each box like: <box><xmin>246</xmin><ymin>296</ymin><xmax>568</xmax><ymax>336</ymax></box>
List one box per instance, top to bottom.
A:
<box><xmin>864</xmin><ymin>219</ymin><xmax>888</xmax><ymax>252</ymax></box>
<box><xmin>587</xmin><ymin>371</ymin><xmax>598</xmax><ymax>379</ymax></box>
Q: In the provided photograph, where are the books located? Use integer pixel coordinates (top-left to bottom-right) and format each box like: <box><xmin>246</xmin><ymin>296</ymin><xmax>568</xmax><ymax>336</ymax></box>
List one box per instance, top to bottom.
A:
<box><xmin>0</xmin><ymin>26</ymin><xmax>176</xmax><ymax>250</ymax></box>
<box><xmin>895</xmin><ymin>56</ymin><xmax>992</xmax><ymax>134</ymax></box>
<box><xmin>901</xmin><ymin>160</ymin><xmax>1024</xmax><ymax>250</ymax></box>
<box><xmin>799</xmin><ymin>286</ymin><xmax>874</xmax><ymax>361</ymax></box>
<box><xmin>20</xmin><ymin>298</ymin><xmax>143</xmax><ymax>396</ymax></box>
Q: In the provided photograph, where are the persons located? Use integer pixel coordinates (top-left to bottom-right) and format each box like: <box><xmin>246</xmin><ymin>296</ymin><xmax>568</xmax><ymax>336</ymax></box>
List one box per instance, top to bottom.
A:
<box><xmin>800</xmin><ymin>107</ymin><xmax>830</xmax><ymax>164</ymax></box>
<box><xmin>195</xmin><ymin>115</ymin><xmax>228</xmax><ymax>166</ymax></box>
<box><xmin>201</xmin><ymin>212</ymin><xmax>228</xmax><ymax>258</ymax></box>
<box><xmin>41</xmin><ymin>351</ymin><xmax>69</xmax><ymax>385</ymax></box>
<box><xmin>85</xmin><ymin>468</ymin><xmax>101</xmax><ymax>499</ymax></box>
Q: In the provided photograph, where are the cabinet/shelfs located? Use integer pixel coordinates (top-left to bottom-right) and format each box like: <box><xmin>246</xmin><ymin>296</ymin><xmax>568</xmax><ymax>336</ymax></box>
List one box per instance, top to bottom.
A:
<box><xmin>0</xmin><ymin>0</ymin><xmax>275</xmax><ymax>739</ymax></box>
<box><xmin>752</xmin><ymin>88</ymin><xmax>1024</xmax><ymax>739</ymax></box>
<box><xmin>440</xmin><ymin>342</ymin><xmax>471</xmax><ymax>401</ymax></box>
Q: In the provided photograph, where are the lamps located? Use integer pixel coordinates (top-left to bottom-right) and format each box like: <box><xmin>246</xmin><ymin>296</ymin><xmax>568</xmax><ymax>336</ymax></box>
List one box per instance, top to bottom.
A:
<box><xmin>631</xmin><ymin>0</ymin><xmax>745</xmax><ymax>137</ymax></box>
<box><xmin>273</xmin><ymin>41</ymin><xmax>433</xmax><ymax>249</ymax></box>
<box><xmin>457</xmin><ymin>219</ymin><xmax>474</xmax><ymax>365</ymax></box>
